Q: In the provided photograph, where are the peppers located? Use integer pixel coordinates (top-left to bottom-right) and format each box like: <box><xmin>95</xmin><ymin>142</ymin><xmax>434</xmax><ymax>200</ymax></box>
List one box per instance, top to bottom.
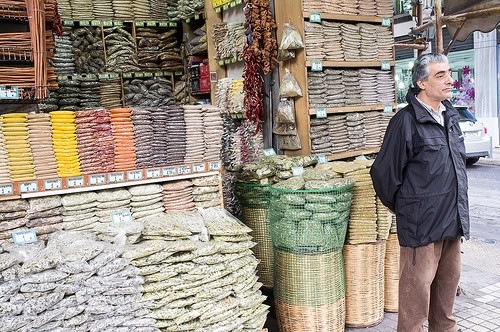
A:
<box><xmin>239</xmin><ymin>43</ymin><xmax>264</xmax><ymax>137</ymax></box>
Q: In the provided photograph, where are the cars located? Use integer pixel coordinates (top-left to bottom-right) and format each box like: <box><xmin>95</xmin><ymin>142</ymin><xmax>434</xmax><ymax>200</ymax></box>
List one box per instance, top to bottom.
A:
<box><xmin>393</xmin><ymin>103</ymin><xmax>493</xmax><ymax>168</ymax></box>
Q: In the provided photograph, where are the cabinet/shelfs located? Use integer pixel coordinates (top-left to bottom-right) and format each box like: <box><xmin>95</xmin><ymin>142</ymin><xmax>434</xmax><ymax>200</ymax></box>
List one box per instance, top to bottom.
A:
<box><xmin>1</xmin><ymin>0</ymin><xmax>398</xmax><ymax>246</ymax></box>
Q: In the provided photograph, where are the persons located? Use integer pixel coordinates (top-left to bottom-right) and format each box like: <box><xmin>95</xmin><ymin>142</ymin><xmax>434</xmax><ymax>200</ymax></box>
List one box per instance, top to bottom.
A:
<box><xmin>368</xmin><ymin>52</ymin><xmax>471</xmax><ymax>332</ymax></box>
